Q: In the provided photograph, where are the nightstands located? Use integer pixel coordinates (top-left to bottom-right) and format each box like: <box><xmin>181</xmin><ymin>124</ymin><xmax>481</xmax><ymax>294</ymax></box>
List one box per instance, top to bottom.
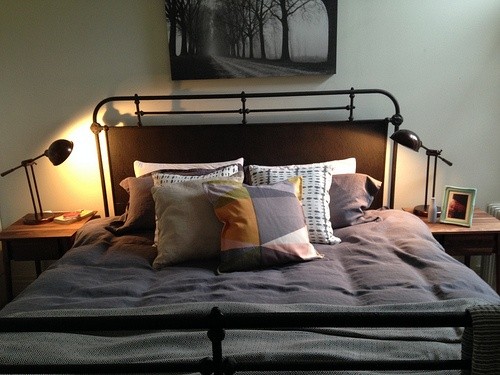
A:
<box><xmin>402</xmin><ymin>206</ymin><xmax>500</xmax><ymax>297</ymax></box>
<box><xmin>0</xmin><ymin>210</ymin><xmax>98</xmax><ymax>302</ymax></box>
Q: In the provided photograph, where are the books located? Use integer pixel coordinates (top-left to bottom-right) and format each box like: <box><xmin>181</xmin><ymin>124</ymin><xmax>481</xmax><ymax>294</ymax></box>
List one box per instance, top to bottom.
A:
<box><xmin>54</xmin><ymin>209</ymin><xmax>93</xmax><ymax>223</ymax></box>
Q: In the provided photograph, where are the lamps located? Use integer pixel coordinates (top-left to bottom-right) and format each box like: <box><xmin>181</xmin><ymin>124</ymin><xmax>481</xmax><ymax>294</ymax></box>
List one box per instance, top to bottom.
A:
<box><xmin>388</xmin><ymin>128</ymin><xmax>453</xmax><ymax>218</ymax></box>
<box><xmin>1</xmin><ymin>139</ymin><xmax>73</xmax><ymax>225</ymax></box>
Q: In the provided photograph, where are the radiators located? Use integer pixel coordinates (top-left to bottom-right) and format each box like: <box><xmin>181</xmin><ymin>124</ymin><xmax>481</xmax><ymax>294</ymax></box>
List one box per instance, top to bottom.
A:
<box><xmin>479</xmin><ymin>202</ymin><xmax>500</xmax><ymax>290</ymax></box>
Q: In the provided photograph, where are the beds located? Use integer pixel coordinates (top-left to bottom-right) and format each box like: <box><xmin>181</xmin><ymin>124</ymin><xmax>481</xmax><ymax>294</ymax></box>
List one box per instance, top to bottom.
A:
<box><xmin>0</xmin><ymin>88</ymin><xmax>500</xmax><ymax>375</ymax></box>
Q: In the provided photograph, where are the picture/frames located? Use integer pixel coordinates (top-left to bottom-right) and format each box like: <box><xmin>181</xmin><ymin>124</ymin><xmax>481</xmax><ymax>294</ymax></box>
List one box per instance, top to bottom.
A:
<box><xmin>439</xmin><ymin>185</ymin><xmax>477</xmax><ymax>229</ymax></box>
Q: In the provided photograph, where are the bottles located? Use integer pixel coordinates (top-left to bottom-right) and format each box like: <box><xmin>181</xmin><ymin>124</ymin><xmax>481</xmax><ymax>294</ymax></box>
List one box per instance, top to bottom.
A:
<box><xmin>427</xmin><ymin>197</ymin><xmax>437</xmax><ymax>223</ymax></box>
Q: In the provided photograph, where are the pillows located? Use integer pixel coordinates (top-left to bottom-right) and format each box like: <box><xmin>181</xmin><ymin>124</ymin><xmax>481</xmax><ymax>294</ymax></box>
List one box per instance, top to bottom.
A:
<box><xmin>328</xmin><ymin>157</ymin><xmax>356</xmax><ymax>175</ymax></box>
<box><xmin>132</xmin><ymin>158</ymin><xmax>244</xmax><ymax>178</ymax></box>
<box><xmin>245</xmin><ymin>161</ymin><xmax>341</xmax><ymax>245</ymax></box>
<box><xmin>105</xmin><ymin>162</ymin><xmax>244</xmax><ymax>237</ymax></box>
<box><xmin>151</xmin><ymin>163</ymin><xmax>239</xmax><ymax>247</ymax></box>
<box><xmin>329</xmin><ymin>173</ymin><xmax>383</xmax><ymax>229</ymax></box>
<box><xmin>202</xmin><ymin>175</ymin><xmax>326</xmax><ymax>273</ymax></box>
<box><xmin>151</xmin><ymin>180</ymin><xmax>223</xmax><ymax>271</ymax></box>
